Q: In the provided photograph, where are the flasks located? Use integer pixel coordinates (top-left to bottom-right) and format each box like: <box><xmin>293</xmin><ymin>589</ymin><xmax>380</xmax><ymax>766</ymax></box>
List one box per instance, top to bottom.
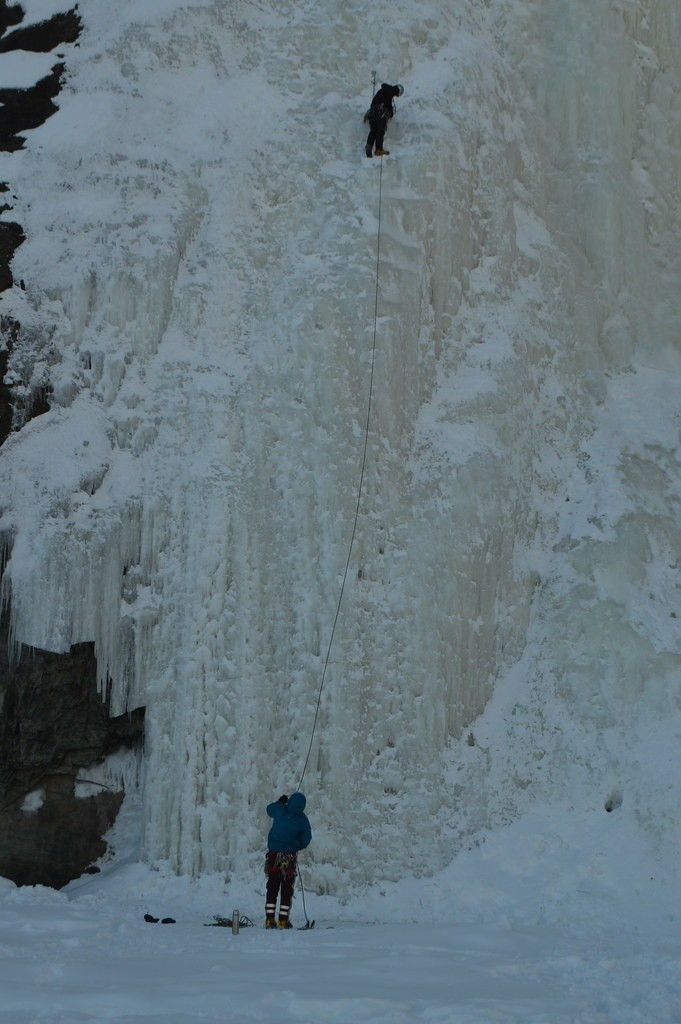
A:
<box><xmin>232</xmin><ymin>910</ymin><xmax>239</xmax><ymax>935</ymax></box>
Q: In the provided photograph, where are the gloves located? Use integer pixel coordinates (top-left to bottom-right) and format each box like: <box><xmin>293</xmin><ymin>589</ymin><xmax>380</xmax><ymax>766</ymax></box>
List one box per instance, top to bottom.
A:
<box><xmin>278</xmin><ymin>794</ymin><xmax>288</xmax><ymax>803</ymax></box>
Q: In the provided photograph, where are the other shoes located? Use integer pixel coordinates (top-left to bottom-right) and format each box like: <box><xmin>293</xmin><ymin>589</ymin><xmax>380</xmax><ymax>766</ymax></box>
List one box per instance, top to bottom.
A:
<box><xmin>365</xmin><ymin>143</ymin><xmax>373</xmax><ymax>157</ymax></box>
<box><xmin>375</xmin><ymin>149</ymin><xmax>389</xmax><ymax>156</ymax></box>
<box><xmin>265</xmin><ymin>919</ymin><xmax>276</xmax><ymax>928</ymax></box>
<box><xmin>277</xmin><ymin>920</ymin><xmax>293</xmax><ymax>929</ymax></box>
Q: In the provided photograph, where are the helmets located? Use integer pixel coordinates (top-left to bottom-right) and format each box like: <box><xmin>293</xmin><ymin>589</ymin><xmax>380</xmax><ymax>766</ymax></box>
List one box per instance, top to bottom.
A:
<box><xmin>396</xmin><ymin>83</ymin><xmax>404</xmax><ymax>98</ymax></box>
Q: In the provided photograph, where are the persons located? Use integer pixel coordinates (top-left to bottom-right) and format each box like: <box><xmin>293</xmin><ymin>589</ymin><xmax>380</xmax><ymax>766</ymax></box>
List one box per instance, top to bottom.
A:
<box><xmin>363</xmin><ymin>83</ymin><xmax>404</xmax><ymax>158</ymax></box>
<box><xmin>264</xmin><ymin>791</ymin><xmax>312</xmax><ymax>929</ymax></box>
